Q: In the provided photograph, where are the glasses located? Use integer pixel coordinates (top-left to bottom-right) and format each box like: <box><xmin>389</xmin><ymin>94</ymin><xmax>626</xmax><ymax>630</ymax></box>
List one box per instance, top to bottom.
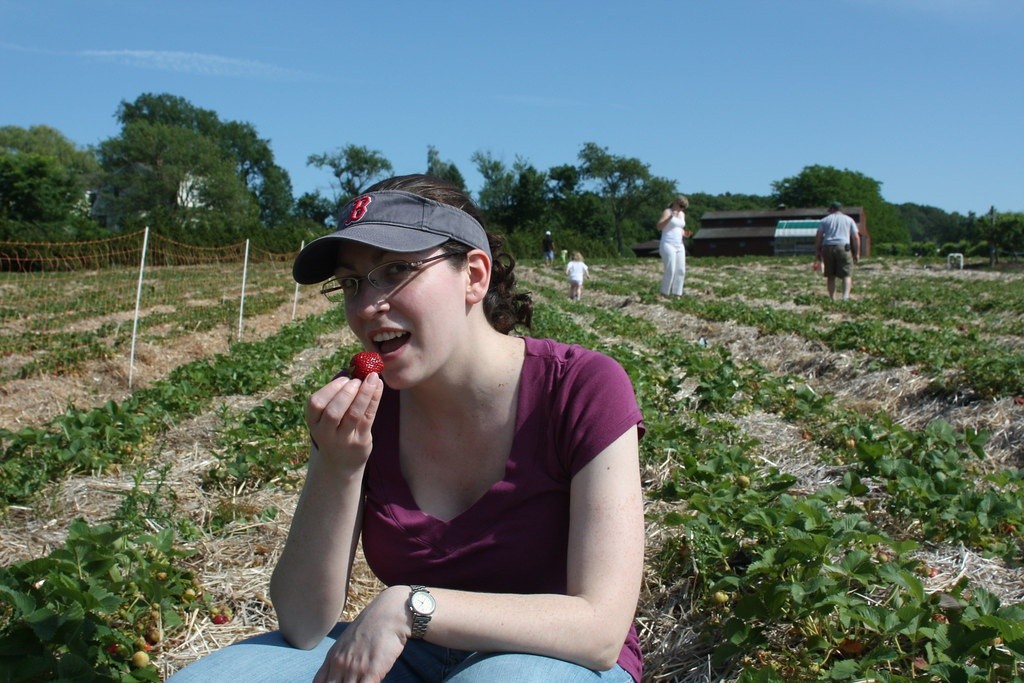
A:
<box><xmin>320</xmin><ymin>249</ymin><xmax>465</xmax><ymax>302</ymax></box>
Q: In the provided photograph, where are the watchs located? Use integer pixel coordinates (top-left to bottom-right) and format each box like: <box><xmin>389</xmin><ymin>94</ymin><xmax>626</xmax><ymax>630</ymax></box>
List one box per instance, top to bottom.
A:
<box><xmin>410</xmin><ymin>584</ymin><xmax>436</xmax><ymax>641</ymax></box>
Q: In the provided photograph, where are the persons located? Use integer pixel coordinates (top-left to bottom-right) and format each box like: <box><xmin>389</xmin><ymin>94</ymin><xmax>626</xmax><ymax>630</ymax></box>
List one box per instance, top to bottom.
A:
<box><xmin>161</xmin><ymin>173</ymin><xmax>648</xmax><ymax>683</ymax></box>
<box><xmin>656</xmin><ymin>194</ymin><xmax>692</xmax><ymax>301</ymax></box>
<box><xmin>814</xmin><ymin>200</ymin><xmax>861</xmax><ymax>303</ymax></box>
<box><xmin>543</xmin><ymin>230</ymin><xmax>555</xmax><ymax>265</ymax></box>
<box><xmin>565</xmin><ymin>250</ymin><xmax>590</xmax><ymax>301</ymax></box>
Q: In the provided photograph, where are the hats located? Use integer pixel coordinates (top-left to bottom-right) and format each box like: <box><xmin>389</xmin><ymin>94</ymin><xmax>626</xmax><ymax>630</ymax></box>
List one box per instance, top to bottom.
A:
<box><xmin>292</xmin><ymin>190</ymin><xmax>492</xmax><ymax>285</ymax></box>
<box><xmin>827</xmin><ymin>202</ymin><xmax>841</xmax><ymax>211</ymax></box>
<box><xmin>546</xmin><ymin>231</ymin><xmax>551</xmax><ymax>235</ymax></box>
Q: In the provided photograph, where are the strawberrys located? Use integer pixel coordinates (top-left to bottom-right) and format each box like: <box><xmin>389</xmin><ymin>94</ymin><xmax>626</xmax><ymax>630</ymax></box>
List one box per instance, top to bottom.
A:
<box><xmin>347</xmin><ymin>351</ymin><xmax>385</xmax><ymax>382</ymax></box>
<box><xmin>711</xmin><ymin>432</ymin><xmax>1015</xmax><ymax>654</ymax></box>
<box><xmin>99</xmin><ymin>433</ymin><xmax>298</xmax><ymax>671</ymax></box>
